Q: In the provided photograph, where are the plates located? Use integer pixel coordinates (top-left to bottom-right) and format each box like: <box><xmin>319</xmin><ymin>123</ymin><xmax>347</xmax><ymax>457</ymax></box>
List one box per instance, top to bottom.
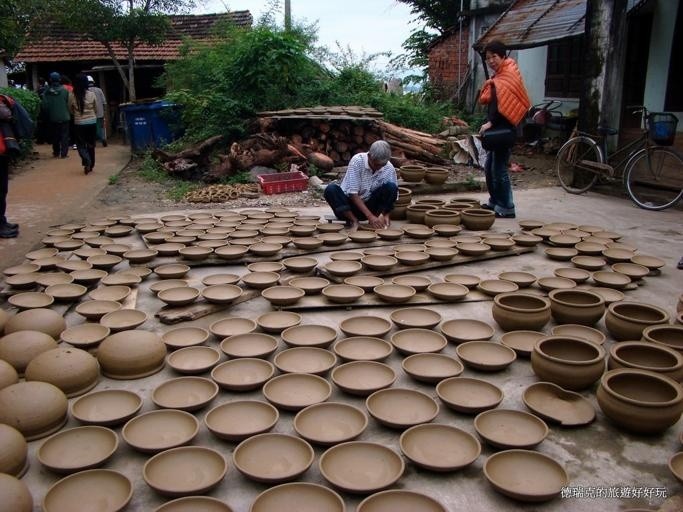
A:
<box><xmin>2</xmin><ymin>207</ymin><xmax>683</xmax><ymax>512</ymax></box>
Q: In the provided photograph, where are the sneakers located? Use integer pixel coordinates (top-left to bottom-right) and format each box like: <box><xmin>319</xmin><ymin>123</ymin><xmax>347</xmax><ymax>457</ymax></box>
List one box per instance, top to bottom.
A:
<box><xmin>0</xmin><ymin>223</ymin><xmax>19</xmax><ymax>229</ymax></box>
<box><xmin>0</xmin><ymin>227</ymin><xmax>19</xmax><ymax>240</ymax></box>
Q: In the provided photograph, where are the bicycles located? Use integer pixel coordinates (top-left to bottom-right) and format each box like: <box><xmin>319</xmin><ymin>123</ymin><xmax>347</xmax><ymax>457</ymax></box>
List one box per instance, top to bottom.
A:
<box><xmin>553</xmin><ymin>102</ymin><xmax>682</xmax><ymax>213</ymax></box>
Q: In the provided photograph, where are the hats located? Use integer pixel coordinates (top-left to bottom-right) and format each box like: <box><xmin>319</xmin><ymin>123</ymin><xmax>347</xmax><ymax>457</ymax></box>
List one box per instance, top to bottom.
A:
<box><xmin>49</xmin><ymin>72</ymin><xmax>61</xmax><ymax>81</ymax></box>
<box><xmin>85</xmin><ymin>75</ymin><xmax>95</xmax><ymax>83</ymax></box>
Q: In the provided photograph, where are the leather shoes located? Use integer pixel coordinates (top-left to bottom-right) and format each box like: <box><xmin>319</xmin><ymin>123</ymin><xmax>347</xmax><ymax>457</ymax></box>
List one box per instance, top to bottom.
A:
<box><xmin>481</xmin><ymin>204</ymin><xmax>494</xmax><ymax>210</ymax></box>
<box><xmin>495</xmin><ymin>211</ymin><xmax>515</xmax><ymax>218</ymax></box>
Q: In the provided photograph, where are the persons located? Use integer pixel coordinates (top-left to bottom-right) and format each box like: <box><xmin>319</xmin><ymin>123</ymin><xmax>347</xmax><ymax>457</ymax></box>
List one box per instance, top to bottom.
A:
<box><xmin>478</xmin><ymin>40</ymin><xmax>530</xmax><ymax>218</ymax></box>
<box><xmin>33</xmin><ymin>71</ymin><xmax>107</xmax><ymax>174</ymax></box>
<box><xmin>0</xmin><ymin>94</ymin><xmax>19</xmax><ymax>239</ymax></box>
<box><xmin>324</xmin><ymin>140</ymin><xmax>400</xmax><ymax>232</ymax></box>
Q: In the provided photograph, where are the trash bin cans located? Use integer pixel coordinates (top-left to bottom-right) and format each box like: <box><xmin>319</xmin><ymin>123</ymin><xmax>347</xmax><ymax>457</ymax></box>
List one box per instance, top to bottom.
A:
<box><xmin>120</xmin><ymin>102</ymin><xmax>152</xmax><ymax>152</ymax></box>
<box><xmin>149</xmin><ymin>102</ymin><xmax>185</xmax><ymax>149</ymax></box>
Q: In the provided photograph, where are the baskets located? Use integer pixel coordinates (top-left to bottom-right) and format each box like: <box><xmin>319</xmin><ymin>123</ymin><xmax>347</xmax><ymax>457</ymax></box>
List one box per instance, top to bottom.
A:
<box><xmin>648</xmin><ymin>111</ymin><xmax>679</xmax><ymax>146</ymax></box>
<box><xmin>257</xmin><ymin>171</ymin><xmax>310</xmax><ymax>195</ymax></box>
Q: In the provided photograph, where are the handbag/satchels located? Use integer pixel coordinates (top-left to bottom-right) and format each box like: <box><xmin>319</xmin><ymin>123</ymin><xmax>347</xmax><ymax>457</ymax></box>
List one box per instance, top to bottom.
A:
<box><xmin>479</xmin><ymin>129</ymin><xmax>514</xmax><ymax>151</ymax></box>
